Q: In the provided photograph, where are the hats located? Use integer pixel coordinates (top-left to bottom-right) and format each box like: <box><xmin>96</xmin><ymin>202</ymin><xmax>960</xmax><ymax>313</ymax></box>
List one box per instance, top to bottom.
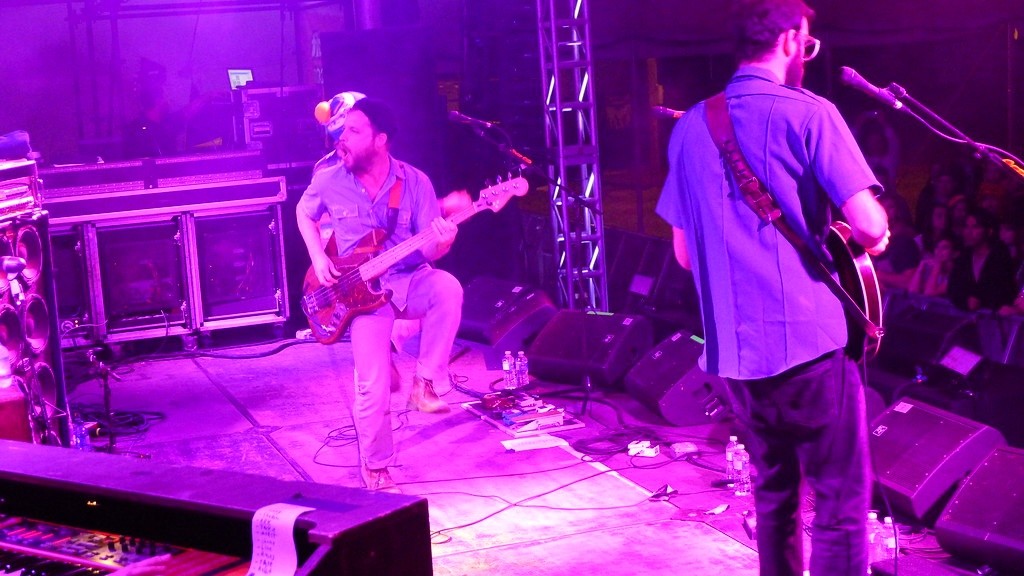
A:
<box><xmin>314</xmin><ymin>92</ymin><xmax>364</xmax><ymax>133</ymax></box>
<box><xmin>354</xmin><ymin>96</ymin><xmax>396</xmax><ymax>139</ymax></box>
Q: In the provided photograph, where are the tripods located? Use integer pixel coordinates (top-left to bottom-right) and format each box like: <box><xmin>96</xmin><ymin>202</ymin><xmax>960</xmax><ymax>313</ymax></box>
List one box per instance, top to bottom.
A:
<box><xmin>468</xmin><ymin>125</ymin><xmax>620</xmax><ymax>415</ymax></box>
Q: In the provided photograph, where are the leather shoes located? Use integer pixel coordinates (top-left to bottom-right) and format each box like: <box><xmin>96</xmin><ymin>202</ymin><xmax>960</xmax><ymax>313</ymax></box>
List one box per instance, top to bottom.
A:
<box><xmin>406</xmin><ymin>376</ymin><xmax>450</xmax><ymax>413</ymax></box>
<box><xmin>360</xmin><ymin>464</ymin><xmax>403</xmax><ymax>496</ymax></box>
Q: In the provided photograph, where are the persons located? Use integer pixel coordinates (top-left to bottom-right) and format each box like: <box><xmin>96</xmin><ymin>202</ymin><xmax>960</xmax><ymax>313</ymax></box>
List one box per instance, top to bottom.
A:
<box><xmin>653</xmin><ymin>0</ymin><xmax>891</xmax><ymax>576</ymax></box>
<box><xmin>293</xmin><ymin>93</ymin><xmax>474</xmax><ymax>499</ymax></box>
<box><xmin>871</xmin><ymin>150</ymin><xmax>1023</xmax><ymax>316</ymax></box>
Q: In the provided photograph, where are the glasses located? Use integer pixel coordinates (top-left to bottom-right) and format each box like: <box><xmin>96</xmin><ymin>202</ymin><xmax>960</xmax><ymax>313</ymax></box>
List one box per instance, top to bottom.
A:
<box><xmin>796</xmin><ymin>30</ymin><xmax>821</xmax><ymax>61</ymax></box>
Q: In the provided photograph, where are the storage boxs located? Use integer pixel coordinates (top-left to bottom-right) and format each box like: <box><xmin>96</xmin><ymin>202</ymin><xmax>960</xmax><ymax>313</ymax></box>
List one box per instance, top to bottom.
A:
<box><xmin>37</xmin><ymin>84</ymin><xmax>326</xmax><ymax>357</ymax></box>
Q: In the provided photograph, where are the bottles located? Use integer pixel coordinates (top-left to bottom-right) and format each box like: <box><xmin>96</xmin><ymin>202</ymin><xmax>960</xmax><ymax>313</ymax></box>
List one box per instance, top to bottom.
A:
<box><xmin>502</xmin><ymin>351</ymin><xmax>517</xmax><ymax>389</ymax></box>
<box><xmin>515</xmin><ymin>351</ymin><xmax>529</xmax><ymax>388</ymax></box>
<box><xmin>72</xmin><ymin>412</ymin><xmax>93</xmax><ymax>453</ymax></box>
<box><xmin>726</xmin><ymin>436</ymin><xmax>739</xmax><ymax>486</ymax></box>
<box><xmin>733</xmin><ymin>444</ymin><xmax>751</xmax><ymax>496</ymax></box>
<box><xmin>866</xmin><ymin>512</ymin><xmax>882</xmax><ymax>574</ymax></box>
<box><xmin>879</xmin><ymin>516</ymin><xmax>899</xmax><ymax>560</ymax></box>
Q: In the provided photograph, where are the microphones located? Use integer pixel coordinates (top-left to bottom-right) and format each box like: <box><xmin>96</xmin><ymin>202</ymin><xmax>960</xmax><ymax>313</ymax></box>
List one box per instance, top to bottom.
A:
<box><xmin>832</xmin><ymin>65</ymin><xmax>910</xmax><ymax>114</ymax></box>
<box><xmin>652</xmin><ymin>107</ymin><xmax>687</xmax><ymax>118</ymax></box>
<box><xmin>448</xmin><ymin>110</ymin><xmax>491</xmax><ymax>127</ymax></box>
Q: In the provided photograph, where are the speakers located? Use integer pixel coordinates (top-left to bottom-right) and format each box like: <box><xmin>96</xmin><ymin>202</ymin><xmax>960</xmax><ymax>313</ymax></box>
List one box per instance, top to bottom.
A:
<box><xmin>1</xmin><ymin>210</ymin><xmax>75</xmax><ymax>447</ymax></box>
<box><xmin>460</xmin><ymin>230</ymin><xmax>1024</xmax><ymax>570</ymax></box>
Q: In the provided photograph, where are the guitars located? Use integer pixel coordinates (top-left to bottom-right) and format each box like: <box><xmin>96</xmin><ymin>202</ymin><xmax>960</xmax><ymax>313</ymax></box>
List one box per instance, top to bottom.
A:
<box><xmin>647</xmin><ymin>102</ymin><xmax>884</xmax><ymax>366</ymax></box>
<box><xmin>300</xmin><ymin>168</ymin><xmax>530</xmax><ymax>346</ymax></box>
